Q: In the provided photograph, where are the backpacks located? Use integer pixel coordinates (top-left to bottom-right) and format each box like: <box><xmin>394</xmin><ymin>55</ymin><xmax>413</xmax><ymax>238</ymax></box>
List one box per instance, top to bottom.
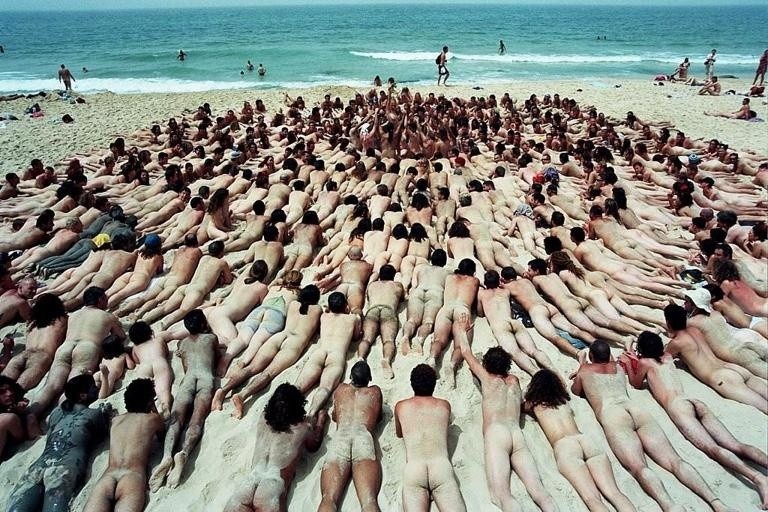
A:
<box><xmin>436</xmin><ymin>52</ymin><xmax>444</xmax><ymax>64</ymax></box>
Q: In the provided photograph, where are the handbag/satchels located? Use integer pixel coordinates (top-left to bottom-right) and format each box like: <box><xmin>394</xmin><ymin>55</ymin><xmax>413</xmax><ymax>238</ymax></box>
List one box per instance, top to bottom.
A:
<box><xmin>441</xmin><ymin>66</ymin><xmax>446</xmax><ymax>73</ymax></box>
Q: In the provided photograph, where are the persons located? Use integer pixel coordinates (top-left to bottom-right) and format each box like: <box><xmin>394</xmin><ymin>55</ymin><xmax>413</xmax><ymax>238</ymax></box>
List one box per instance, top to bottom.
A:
<box><xmin>498</xmin><ymin>39</ymin><xmax>506</xmax><ymax>55</ymax></box>
<box><xmin>0</xmin><ymin>45</ymin><xmax>4</xmax><ymax>53</ymax></box>
<box><xmin>437</xmin><ymin>45</ymin><xmax>450</xmax><ymax>86</ymax></box>
<box><xmin>702</xmin><ymin>49</ymin><xmax>717</xmax><ymax>81</ymax></box>
<box><xmin>752</xmin><ymin>49</ymin><xmax>768</xmax><ymax>87</ymax></box>
<box><xmin>177</xmin><ymin>50</ymin><xmax>187</xmax><ymax>61</ymax></box>
<box><xmin>671</xmin><ymin>57</ymin><xmax>690</xmax><ymax>77</ymax></box>
<box><xmin>246</xmin><ymin>60</ymin><xmax>254</xmax><ymax>71</ymax></box>
<box><xmin>257</xmin><ymin>63</ymin><xmax>266</xmax><ymax>76</ymax></box>
<box><xmin>57</xmin><ymin>64</ymin><xmax>76</xmax><ymax>91</ymax></box>
<box><xmin>82</xmin><ymin>68</ymin><xmax>87</xmax><ymax>73</ymax></box>
<box><xmin>0</xmin><ymin>71</ymin><xmax>768</xmax><ymax>512</ymax></box>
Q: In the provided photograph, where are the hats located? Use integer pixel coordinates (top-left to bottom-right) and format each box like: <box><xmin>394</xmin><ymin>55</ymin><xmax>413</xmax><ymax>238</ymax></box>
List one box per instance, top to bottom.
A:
<box><xmin>230</xmin><ymin>152</ymin><xmax>240</xmax><ymax>159</ymax></box>
<box><xmin>689</xmin><ymin>154</ymin><xmax>699</xmax><ymax>163</ymax></box>
<box><xmin>356</xmin><ymin>161</ymin><xmax>364</xmax><ymax>166</ymax></box>
<box><xmin>533</xmin><ymin>174</ymin><xmax>546</xmax><ymax>184</ymax></box>
<box><xmin>145</xmin><ymin>234</ymin><xmax>166</xmax><ymax>247</ymax></box>
<box><xmin>684</xmin><ymin>288</ymin><xmax>714</xmax><ymax>314</ymax></box>
